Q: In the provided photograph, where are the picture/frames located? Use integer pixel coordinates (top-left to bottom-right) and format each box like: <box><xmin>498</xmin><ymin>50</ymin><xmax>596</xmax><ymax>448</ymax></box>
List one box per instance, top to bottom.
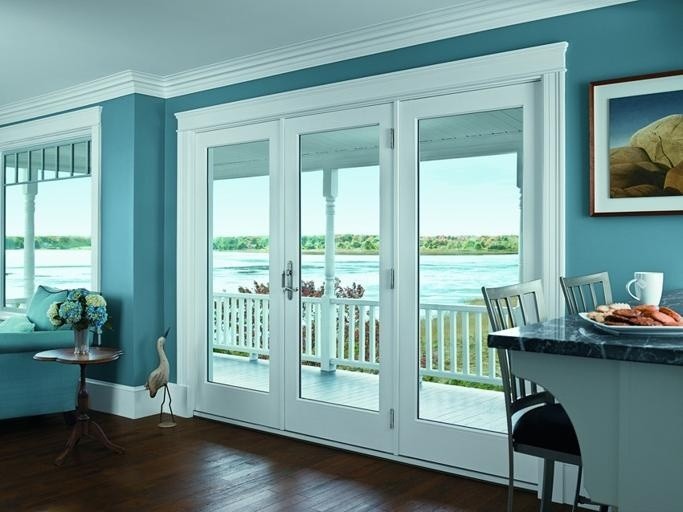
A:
<box><xmin>587</xmin><ymin>69</ymin><xmax>683</xmax><ymax>218</ymax></box>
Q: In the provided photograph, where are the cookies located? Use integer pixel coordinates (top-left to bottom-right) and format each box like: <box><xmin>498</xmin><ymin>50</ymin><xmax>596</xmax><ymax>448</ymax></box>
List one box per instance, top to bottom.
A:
<box><xmin>588</xmin><ymin>302</ymin><xmax>681</xmax><ymax>326</ymax></box>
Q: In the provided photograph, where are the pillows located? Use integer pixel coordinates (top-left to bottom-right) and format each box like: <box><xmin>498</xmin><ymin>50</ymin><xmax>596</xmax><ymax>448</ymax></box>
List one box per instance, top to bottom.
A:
<box><xmin>0</xmin><ymin>286</ymin><xmax>69</xmax><ymax>333</ymax></box>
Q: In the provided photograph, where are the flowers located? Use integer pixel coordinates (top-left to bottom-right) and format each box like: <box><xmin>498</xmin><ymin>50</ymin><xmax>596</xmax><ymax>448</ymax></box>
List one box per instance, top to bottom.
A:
<box><xmin>47</xmin><ymin>287</ymin><xmax>115</xmax><ymax>334</ymax></box>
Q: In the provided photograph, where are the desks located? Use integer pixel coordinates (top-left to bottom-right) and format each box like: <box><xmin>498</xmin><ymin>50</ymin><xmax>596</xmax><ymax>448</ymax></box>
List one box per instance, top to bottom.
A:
<box><xmin>33</xmin><ymin>346</ymin><xmax>128</xmax><ymax>465</ymax></box>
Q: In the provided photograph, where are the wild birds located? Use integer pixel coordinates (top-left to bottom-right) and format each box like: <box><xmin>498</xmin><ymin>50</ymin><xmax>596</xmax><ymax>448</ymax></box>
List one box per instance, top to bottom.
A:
<box><xmin>144</xmin><ymin>326</ymin><xmax>176</xmax><ymax>422</ymax></box>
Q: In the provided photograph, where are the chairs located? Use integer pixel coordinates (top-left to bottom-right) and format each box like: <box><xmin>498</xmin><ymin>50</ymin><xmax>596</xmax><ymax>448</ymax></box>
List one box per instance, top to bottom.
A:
<box><xmin>482</xmin><ymin>271</ymin><xmax>615</xmax><ymax>512</ymax></box>
<box><xmin>0</xmin><ymin>284</ymin><xmax>103</xmax><ymax>427</ymax></box>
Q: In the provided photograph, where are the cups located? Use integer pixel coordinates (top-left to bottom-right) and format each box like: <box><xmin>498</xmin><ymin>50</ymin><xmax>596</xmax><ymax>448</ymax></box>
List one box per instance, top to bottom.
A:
<box><xmin>626</xmin><ymin>272</ymin><xmax>664</xmax><ymax>309</ymax></box>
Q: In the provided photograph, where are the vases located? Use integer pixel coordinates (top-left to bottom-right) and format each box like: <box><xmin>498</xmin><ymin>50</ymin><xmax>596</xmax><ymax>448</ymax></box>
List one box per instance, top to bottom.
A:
<box><xmin>73</xmin><ymin>326</ymin><xmax>90</xmax><ymax>354</ymax></box>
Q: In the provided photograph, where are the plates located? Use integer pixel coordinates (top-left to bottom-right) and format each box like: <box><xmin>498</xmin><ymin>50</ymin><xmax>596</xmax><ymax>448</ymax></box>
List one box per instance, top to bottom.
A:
<box><xmin>580</xmin><ymin>311</ymin><xmax>682</xmax><ymax>336</ymax></box>
